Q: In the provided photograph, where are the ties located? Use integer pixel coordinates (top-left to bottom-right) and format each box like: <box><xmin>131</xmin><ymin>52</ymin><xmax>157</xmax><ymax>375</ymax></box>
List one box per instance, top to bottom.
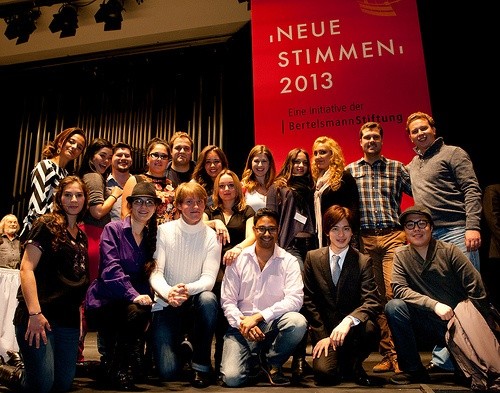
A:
<box><xmin>332</xmin><ymin>256</ymin><xmax>341</xmax><ymax>285</ymax></box>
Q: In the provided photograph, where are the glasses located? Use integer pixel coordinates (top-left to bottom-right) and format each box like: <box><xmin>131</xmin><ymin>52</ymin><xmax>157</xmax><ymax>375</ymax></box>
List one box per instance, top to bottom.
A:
<box><xmin>150</xmin><ymin>153</ymin><xmax>168</xmax><ymax>160</ymax></box>
<box><xmin>134</xmin><ymin>199</ymin><xmax>155</xmax><ymax>207</ymax></box>
<box><xmin>405</xmin><ymin>219</ymin><xmax>428</xmax><ymax>230</ymax></box>
<box><xmin>255</xmin><ymin>226</ymin><xmax>278</xmax><ymax>233</ymax></box>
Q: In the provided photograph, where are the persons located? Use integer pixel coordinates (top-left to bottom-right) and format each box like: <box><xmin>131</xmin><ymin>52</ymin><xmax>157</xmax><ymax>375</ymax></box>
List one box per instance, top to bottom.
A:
<box><xmin>403</xmin><ymin>113</ymin><xmax>483</xmax><ymax>372</ymax></box>
<box><xmin>340</xmin><ymin>123</ymin><xmax>415</xmax><ymax>374</ymax></box>
<box><xmin>18</xmin><ymin>127</ymin><xmax>86</xmax><ymax>249</ymax></box>
<box><xmin>166</xmin><ymin>131</ymin><xmax>199</xmax><ymax>189</ymax></box>
<box><xmin>310</xmin><ymin>136</ymin><xmax>360</xmax><ymax>250</ymax></box>
<box><xmin>77</xmin><ymin>137</ymin><xmax>116</xmax><ymax>372</ymax></box>
<box><xmin>120</xmin><ymin>138</ymin><xmax>180</xmax><ymax>384</ymax></box>
<box><xmin>105</xmin><ymin>142</ymin><xmax>135</xmax><ymax>221</ymax></box>
<box><xmin>238</xmin><ymin>143</ymin><xmax>277</xmax><ymax>216</ymax></box>
<box><xmin>189</xmin><ymin>145</ymin><xmax>230</xmax><ymax>214</ymax></box>
<box><xmin>264</xmin><ymin>147</ymin><xmax>319</xmax><ymax>376</ymax></box>
<box><xmin>209</xmin><ymin>168</ymin><xmax>261</xmax><ymax>267</ymax></box>
<box><xmin>12</xmin><ymin>175</ymin><xmax>91</xmax><ymax>393</ymax></box>
<box><xmin>85</xmin><ymin>180</ymin><xmax>162</xmax><ymax>391</ymax></box>
<box><xmin>148</xmin><ymin>183</ymin><xmax>222</xmax><ymax>388</ymax></box>
<box><xmin>303</xmin><ymin>205</ymin><xmax>384</xmax><ymax>387</ymax></box>
<box><xmin>384</xmin><ymin>207</ymin><xmax>486</xmax><ymax>387</ymax></box>
<box><xmin>220</xmin><ymin>208</ymin><xmax>307</xmax><ymax>387</ymax></box>
<box><xmin>0</xmin><ymin>214</ymin><xmax>23</xmax><ymax>269</ymax></box>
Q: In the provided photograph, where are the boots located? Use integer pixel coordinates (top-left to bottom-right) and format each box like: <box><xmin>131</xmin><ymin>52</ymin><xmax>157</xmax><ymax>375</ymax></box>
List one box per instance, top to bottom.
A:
<box><xmin>0</xmin><ymin>351</ymin><xmax>24</xmax><ymax>393</ymax></box>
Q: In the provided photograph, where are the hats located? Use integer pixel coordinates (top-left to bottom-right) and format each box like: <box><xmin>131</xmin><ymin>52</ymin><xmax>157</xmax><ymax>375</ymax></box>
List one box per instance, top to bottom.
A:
<box><xmin>126</xmin><ymin>182</ymin><xmax>162</xmax><ymax>205</ymax></box>
<box><xmin>399</xmin><ymin>205</ymin><xmax>434</xmax><ymax>226</ymax></box>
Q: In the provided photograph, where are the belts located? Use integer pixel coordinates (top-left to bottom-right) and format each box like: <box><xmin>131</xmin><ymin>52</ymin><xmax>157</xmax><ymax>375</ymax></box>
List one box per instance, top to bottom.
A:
<box><xmin>361</xmin><ymin>227</ymin><xmax>397</xmax><ymax>235</ymax></box>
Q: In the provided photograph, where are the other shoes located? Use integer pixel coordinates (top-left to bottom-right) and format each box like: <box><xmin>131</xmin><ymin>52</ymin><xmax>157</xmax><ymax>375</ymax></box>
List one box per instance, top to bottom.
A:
<box><xmin>181</xmin><ymin>341</ymin><xmax>193</xmax><ymax>381</ymax></box>
<box><xmin>373</xmin><ymin>356</ymin><xmax>393</xmax><ymax>371</ymax></box>
<box><xmin>292</xmin><ymin>359</ymin><xmax>313</xmax><ymax>381</ymax></box>
<box><xmin>102</xmin><ymin>360</ymin><xmax>112</xmax><ymax>372</ymax></box>
<box><xmin>393</xmin><ymin>360</ymin><xmax>402</xmax><ymax>372</ymax></box>
<box><xmin>261</xmin><ymin>361</ymin><xmax>290</xmax><ymax>385</ymax></box>
<box><xmin>352</xmin><ymin>363</ymin><xmax>374</xmax><ymax>386</ymax></box>
<box><xmin>108</xmin><ymin>365</ymin><xmax>133</xmax><ymax>390</ymax></box>
<box><xmin>193</xmin><ymin>370</ymin><xmax>207</xmax><ymax>387</ymax></box>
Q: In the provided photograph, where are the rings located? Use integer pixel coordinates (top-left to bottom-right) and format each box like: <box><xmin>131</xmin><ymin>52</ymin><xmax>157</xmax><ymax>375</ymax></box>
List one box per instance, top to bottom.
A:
<box><xmin>219</xmin><ymin>232</ymin><xmax>224</xmax><ymax>235</ymax></box>
<box><xmin>230</xmin><ymin>254</ymin><xmax>233</xmax><ymax>258</ymax></box>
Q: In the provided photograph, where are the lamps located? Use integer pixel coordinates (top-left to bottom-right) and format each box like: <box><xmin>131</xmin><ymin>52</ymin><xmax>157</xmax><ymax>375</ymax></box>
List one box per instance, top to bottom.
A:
<box><xmin>4</xmin><ymin>0</ymin><xmax>126</xmax><ymax>45</ymax></box>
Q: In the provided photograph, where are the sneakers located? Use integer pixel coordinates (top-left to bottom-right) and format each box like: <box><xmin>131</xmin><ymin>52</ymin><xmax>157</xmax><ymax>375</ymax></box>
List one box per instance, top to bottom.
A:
<box><xmin>390</xmin><ymin>366</ymin><xmax>430</xmax><ymax>384</ymax></box>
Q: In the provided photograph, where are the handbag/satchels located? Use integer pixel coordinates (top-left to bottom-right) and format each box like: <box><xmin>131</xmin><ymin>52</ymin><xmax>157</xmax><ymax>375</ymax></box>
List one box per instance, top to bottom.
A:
<box><xmin>442</xmin><ymin>299</ymin><xmax>500</xmax><ymax>393</ymax></box>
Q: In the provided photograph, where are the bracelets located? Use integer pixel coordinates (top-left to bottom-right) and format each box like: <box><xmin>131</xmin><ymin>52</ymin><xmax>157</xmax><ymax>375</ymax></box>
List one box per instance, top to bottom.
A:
<box><xmin>108</xmin><ymin>193</ymin><xmax>118</xmax><ymax>202</ymax></box>
<box><xmin>234</xmin><ymin>245</ymin><xmax>244</xmax><ymax>251</ymax></box>
<box><xmin>29</xmin><ymin>312</ymin><xmax>42</xmax><ymax>316</ymax></box>
